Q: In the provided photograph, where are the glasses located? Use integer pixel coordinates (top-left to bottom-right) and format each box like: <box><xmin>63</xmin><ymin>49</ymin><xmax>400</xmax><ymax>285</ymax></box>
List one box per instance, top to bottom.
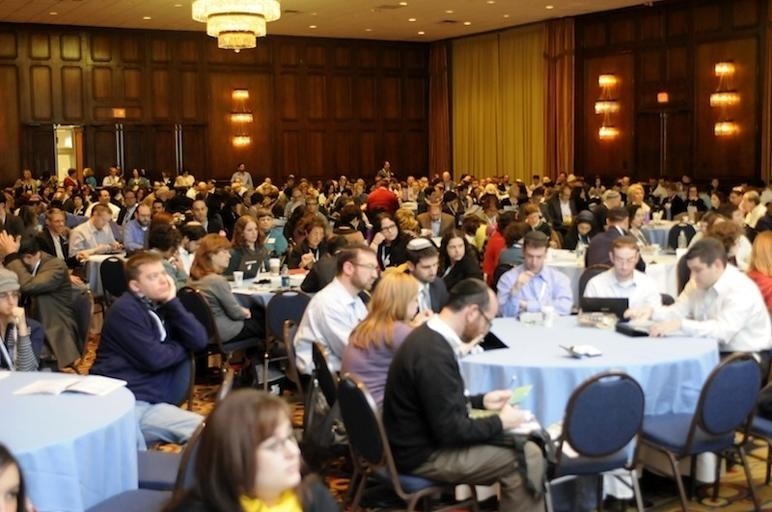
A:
<box><xmin>353</xmin><ymin>262</ymin><xmax>380</xmax><ymax>271</ymax></box>
<box><xmin>148</xmin><ymin>269</ymin><xmax>168</xmax><ymax>281</ymax></box>
<box><xmin>0</xmin><ymin>291</ymin><xmax>20</xmax><ymax>299</ymax></box>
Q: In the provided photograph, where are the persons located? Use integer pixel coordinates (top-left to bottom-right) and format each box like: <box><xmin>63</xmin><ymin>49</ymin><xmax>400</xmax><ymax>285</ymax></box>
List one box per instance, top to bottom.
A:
<box><xmin>540</xmin><ymin>159</ymin><xmax>772</xmax><ymax>228</ymax></box>
<box><xmin>211</xmin><ymin>161</ymin><xmax>415</xmax><ymax>234</ymax></box>
<box><xmin>0</xmin><ymin>165</ymin><xmax>210</xmax><ymax>231</ymax></box>
<box><xmin>416</xmin><ymin>171</ymin><xmax>541</xmax><ymax>237</ymax></box>
<box><xmin>123</xmin><ymin>199</ymin><xmax>151</xmax><ymax>254</ymax></box>
<box><xmin>69</xmin><ymin>203</ymin><xmax>121</xmax><ymax>259</ymax></box>
<box><xmin>37</xmin><ymin>206</ymin><xmax>76</xmax><ymax>271</ymax></box>
<box><xmin>584</xmin><ymin>207</ymin><xmax>646</xmax><ymax>273</ymax></box>
<box><xmin>253</xmin><ymin>208</ymin><xmax>287</xmax><ymax>258</ymax></box>
<box><xmin>564</xmin><ymin>211</ymin><xmax>592</xmax><ymax>249</ymax></box>
<box><xmin>367</xmin><ymin>212</ymin><xmax>416</xmax><ymax>271</ymax></box>
<box><xmin>223</xmin><ymin>215</ymin><xmax>270</xmax><ymax>280</ymax></box>
<box><xmin>287</xmin><ymin>222</ymin><xmax>327</xmax><ymax>268</ymax></box>
<box><xmin>147</xmin><ymin>224</ymin><xmax>188</xmax><ymax>291</ymax></box>
<box><xmin>495</xmin><ymin>224</ymin><xmax>527</xmax><ymax>284</ymax></box>
<box><xmin>436</xmin><ymin>228</ymin><xmax>481</xmax><ymax>292</ymax></box>
<box><xmin>0</xmin><ymin>229</ymin><xmax>83</xmax><ymax>372</ymax></box>
<box><xmin>745</xmin><ymin>230</ymin><xmax>772</xmax><ymax>319</ymax></box>
<box><xmin>495</xmin><ymin>231</ymin><xmax>574</xmax><ymax>317</ymax></box>
<box><xmin>187</xmin><ymin>234</ymin><xmax>265</xmax><ymax>347</ymax></box>
<box><xmin>301</xmin><ymin>235</ymin><xmax>348</xmax><ymax>291</ymax></box>
<box><xmin>578</xmin><ymin>235</ymin><xmax>662</xmax><ymax>315</ymax></box>
<box><xmin>623</xmin><ymin>237</ymin><xmax>771</xmax><ymax>391</ymax></box>
<box><xmin>407</xmin><ymin>239</ymin><xmax>448</xmax><ymax>321</ymax></box>
<box><xmin>292</xmin><ymin>244</ymin><xmax>378</xmax><ymax>378</ymax></box>
<box><xmin>86</xmin><ymin>252</ymin><xmax>205</xmax><ymax>454</ymax></box>
<box><xmin>0</xmin><ymin>269</ymin><xmax>44</xmax><ymax>372</ymax></box>
<box><xmin>341</xmin><ymin>272</ymin><xmax>433</xmax><ymax>419</ymax></box>
<box><xmin>382</xmin><ymin>278</ymin><xmax>547</xmax><ymax>511</ymax></box>
<box><xmin>175</xmin><ymin>389</ymin><xmax>339</xmax><ymax>512</ymax></box>
<box><xmin>0</xmin><ymin>441</ymin><xmax>37</xmax><ymax>512</ymax></box>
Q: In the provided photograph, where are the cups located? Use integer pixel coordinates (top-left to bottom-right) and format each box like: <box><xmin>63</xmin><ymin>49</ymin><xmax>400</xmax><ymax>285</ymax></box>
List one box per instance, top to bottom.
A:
<box><xmin>676</xmin><ymin>248</ymin><xmax>686</xmax><ymax>259</ymax></box>
<box><xmin>269</xmin><ymin>259</ymin><xmax>280</xmax><ymax>278</ymax></box>
<box><xmin>233</xmin><ymin>271</ymin><xmax>243</xmax><ymax>288</ymax></box>
<box><xmin>541</xmin><ymin>307</ymin><xmax>553</xmax><ymax>325</ymax></box>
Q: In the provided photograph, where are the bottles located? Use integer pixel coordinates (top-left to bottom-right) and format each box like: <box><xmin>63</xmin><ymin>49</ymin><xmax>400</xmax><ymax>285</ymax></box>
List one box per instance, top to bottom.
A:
<box><xmin>678</xmin><ymin>230</ymin><xmax>687</xmax><ymax>249</ymax></box>
<box><xmin>575</xmin><ymin>240</ymin><xmax>585</xmax><ymax>268</ymax></box>
<box><xmin>281</xmin><ymin>264</ymin><xmax>290</xmax><ymax>288</ymax></box>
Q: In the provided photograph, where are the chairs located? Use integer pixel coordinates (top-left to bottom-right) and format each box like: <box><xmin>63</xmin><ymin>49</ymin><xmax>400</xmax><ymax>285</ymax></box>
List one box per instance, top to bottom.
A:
<box><xmin>100</xmin><ymin>256</ymin><xmax>129</xmax><ymax>307</ymax></box>
<box><xmin>492</xmin><ymin>263</ymin><xmax>513</xmax><ymax>293</ymax></box>
<box><xmin>578</xmin><ymin>264</ymin><xmax>610</xmax><ymax>308</ymax></box>
<box><xmin>176</xmin><ymin>286</ymin><xmax>263</xmax><ymax>361</ymax></box>
<box><xmin>263</xmin><ymin>289</ymin><xmax>312</xmax><ymax>393</ymax></box>
<box><xmin>72</xmin><ymin>290</ymin><xmax>91</xmax><ymax>357</ymax></box>
<box><xmin>302</xmin><ymin>342</ymin><xmax>339</xmax><ymax>439</ymax></box>
<box><xmin>640</xmin><ymin>350</ymin><xmax>763</xmax><ymax>512</ymax></box>
<box><xmin>178</xmin><ymin>354</ymin><xmax>197</xmax><ymax>410</ymax></box>
<box><xmin>738</xmin><ymin>364</ymin><xmax>772</xmax><ymax>484</ymax></box>
<box><xmin>137</xmin><ymin>365</ymin><xmax>235</xmax><ymax>489</ymax></box>
<box><xmin>337</xmin><ymin>372</ymin><xmax>479</xmax><ymax>512</ymax></box>
<box><xmin>542</xmin><ymin>372</ymin><xmax>647</xmax><ymax>512</ymax></box>
<box><xmin>85</xmin><ymin>422</ymin><xmax>206</xmax><ymax>511</ymax></box>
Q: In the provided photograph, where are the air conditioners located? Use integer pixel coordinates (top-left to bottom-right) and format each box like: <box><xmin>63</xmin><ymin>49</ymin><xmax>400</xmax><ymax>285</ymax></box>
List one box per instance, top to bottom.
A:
<box><xmin>54</xmin><ymin>124</ymin><xmax>83</xmax><ymax>181</ymax></box>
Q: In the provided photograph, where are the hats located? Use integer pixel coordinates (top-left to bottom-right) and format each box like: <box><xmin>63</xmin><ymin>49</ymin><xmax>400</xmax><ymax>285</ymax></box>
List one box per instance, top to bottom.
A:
<box><xmin>577</xmin><ymin>210</ymin><xmax>594</xmax><ymax>222</ymax></box>
<box><xmin>406</xmin><ymin>238</ymin><xmax>432</xmax><ymax>251</ymax></box>
<box><xmin>0</xmin><ymin>268</ymin><xmax>20</xmax><ymax>292</ymax></box>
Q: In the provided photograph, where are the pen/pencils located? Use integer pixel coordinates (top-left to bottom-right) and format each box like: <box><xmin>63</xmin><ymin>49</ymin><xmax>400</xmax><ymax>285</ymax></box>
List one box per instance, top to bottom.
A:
<box><xmin>502</xmin><ymin>375</ymin><xmax>519</xmax><ymax>391</ymax></box>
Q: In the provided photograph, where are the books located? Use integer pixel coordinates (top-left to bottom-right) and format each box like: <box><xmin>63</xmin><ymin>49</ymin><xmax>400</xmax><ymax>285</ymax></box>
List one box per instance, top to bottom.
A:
<box><xmin>13</xmin><ymin>375</ymin><xmax>127</xmax><ymax>396</ymax></box>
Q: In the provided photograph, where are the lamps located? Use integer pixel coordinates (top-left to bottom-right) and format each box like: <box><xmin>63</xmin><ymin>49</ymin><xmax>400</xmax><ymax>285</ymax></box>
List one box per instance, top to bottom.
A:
<box><xmin>191</xmin><ymin>0</ymin><xmax>282</xmax><ymax>54</ymax></box>
<box><xmin>710</xmin><ymin>61</ymin><xmax>741</xmax><ymax>138</ymax></box>
<box><xmin>593</xmin><ymin>73</ymin><xmax>620</xmax><ymax>142</ymax></box>
<box><xmin>230</xmin><ymin>88</ymin><xmax>254</xmax><ymax>148</ymax></box>
